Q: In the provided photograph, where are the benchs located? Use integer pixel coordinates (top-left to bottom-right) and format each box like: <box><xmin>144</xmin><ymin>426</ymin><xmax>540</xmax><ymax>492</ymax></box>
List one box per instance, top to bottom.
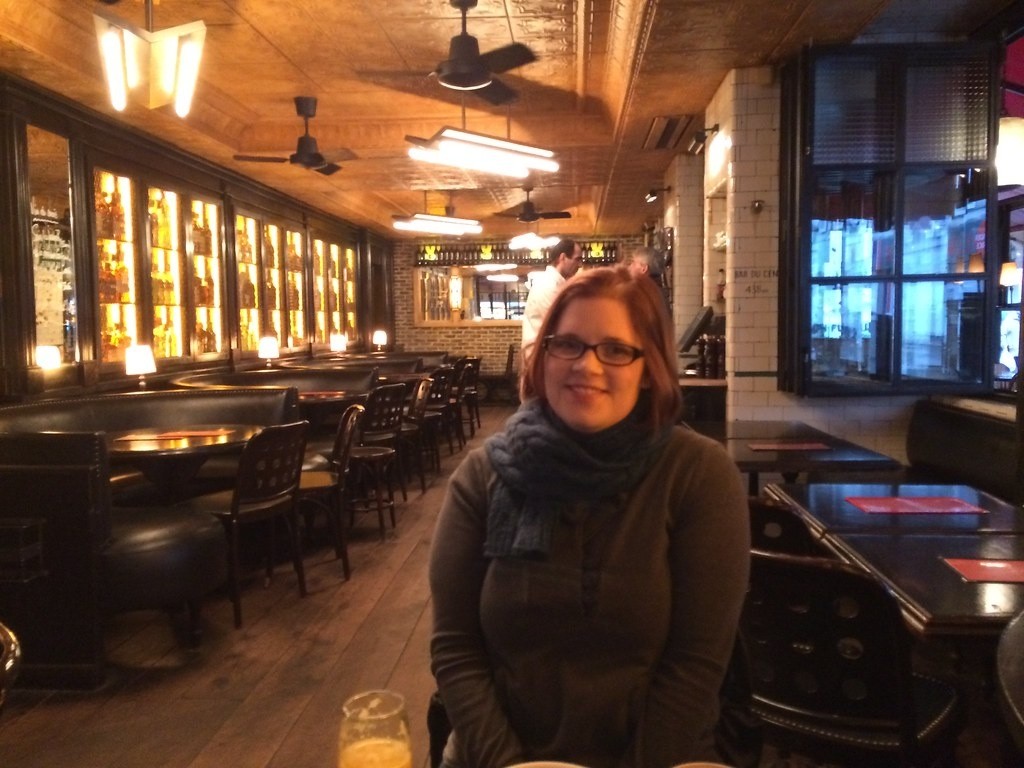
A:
<box><xmin>0</xmin><ymin>343</ymin><xmax>464</xmax><ymax>694</ymax></box>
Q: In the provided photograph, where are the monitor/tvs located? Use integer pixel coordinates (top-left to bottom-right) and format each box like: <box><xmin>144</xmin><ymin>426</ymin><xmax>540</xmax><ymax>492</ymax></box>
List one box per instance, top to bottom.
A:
<box><xmin>676</xmin><ymin>306</ymin><xmax>714</xmax><ymax>353</ymax></box>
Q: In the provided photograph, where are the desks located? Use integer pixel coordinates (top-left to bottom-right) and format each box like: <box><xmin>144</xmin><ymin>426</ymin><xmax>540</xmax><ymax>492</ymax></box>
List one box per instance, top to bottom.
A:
<box><xmin>378</xmin><ymin>372</ymin><xmax>427</xmax><ymax>403</ymax></box>
<box><xmin>676</xmin><ymin>378</ymin><xmax>1024</xmax><ymax>751</ymax></box>
<box><xmin>301</xmin><ymin>390</ymin><xmax>369</xmax><ymax>442</ymax></box>
<box><xmin>102</xmin><ymin>424</ymin><xmax>264</xmax><ymax>515</ymax></box>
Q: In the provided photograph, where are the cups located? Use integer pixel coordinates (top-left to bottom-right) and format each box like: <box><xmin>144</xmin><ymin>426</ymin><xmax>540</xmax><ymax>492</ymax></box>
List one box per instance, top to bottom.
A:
<box><xmin>338</xmin><ymin>689</ymin><xmax>411</xmax><ymax>767</ymax></box>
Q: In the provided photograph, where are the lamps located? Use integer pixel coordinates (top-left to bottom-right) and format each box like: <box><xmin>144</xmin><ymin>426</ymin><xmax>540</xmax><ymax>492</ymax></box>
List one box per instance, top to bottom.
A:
<box><xmin>93</xmin><ymin>0</ymin><xmax>206</xmax><ymax>117</ymax></box>
<box><xmin>407</xmin><ymin>91</ymin><xmax>560</xmax><ymax>177</ymax></box>
<box><xmin>687</xmin><ymin>124</ymin><xmax>719</xmax><ymax>154</ymax></box>
<box><xmin>644</xmin><ymin>186</ymin><xmax>671</xmax><ymax>202</ymax></box>
<box><xmin>392</xmin><ymin>189</ymin><xmax>483</xmax><ymax>237</ymax></box>
<box><xmin>968</xmin><ymin>253</ymin><xmax>985</xmax><ymax>292</ymax></box>
<box><xmin>998</xmin><ymin>263</ymin><xmax>1022</xmax><ymax>303</ymax></box>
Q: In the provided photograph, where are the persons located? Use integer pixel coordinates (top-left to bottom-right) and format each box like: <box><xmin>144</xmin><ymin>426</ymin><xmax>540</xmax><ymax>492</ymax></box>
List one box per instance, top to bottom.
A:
<box><xmin>517</xmin><ymin>239</ymin><xmax>583</xmax><ymax>405</ymax></box>
<box><xmin>627</xmin><ymin>247</ymin><xmax>671</xmax><ymax>316</ymax></box>
<box><xmin>426</xmin><ymin>264</ymin><xmax>752</xmax><ymax>768</ymax></box>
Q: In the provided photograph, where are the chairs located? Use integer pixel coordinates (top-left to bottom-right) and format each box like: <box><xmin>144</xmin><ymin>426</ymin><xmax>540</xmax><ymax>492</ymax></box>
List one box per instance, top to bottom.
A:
<box><xmin>170</xmin><ymin>354</ymin><xmax>482</xmax><ymax>631</ymax></box>
<box><xmin>719</xmin><ymin>505</ymin><xmax>974</xmax><ymax>768</ymax></box>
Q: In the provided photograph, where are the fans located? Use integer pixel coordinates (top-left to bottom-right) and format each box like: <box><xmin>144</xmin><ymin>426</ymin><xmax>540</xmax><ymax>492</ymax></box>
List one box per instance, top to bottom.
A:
<box><xmin>354</xmin><ymin>0</ymin><xmax>540</xmax><ymax>107</ymax></box>
<box><xmin>233</xmin><ymin>96</ymin><xmax>362</xmax><ymax>177</ymax></box>
<box><xmin>493</xmin><ymin>186</ymin><xmax>571</xmax><ymax>222</ymax></box>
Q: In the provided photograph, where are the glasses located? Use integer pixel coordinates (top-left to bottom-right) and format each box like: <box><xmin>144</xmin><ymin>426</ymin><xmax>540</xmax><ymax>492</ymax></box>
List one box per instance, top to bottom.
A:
<box><xmin>541</xmin><ymin>335</ymin><xmax>645</xmax><ymax>366</ymax></box>
